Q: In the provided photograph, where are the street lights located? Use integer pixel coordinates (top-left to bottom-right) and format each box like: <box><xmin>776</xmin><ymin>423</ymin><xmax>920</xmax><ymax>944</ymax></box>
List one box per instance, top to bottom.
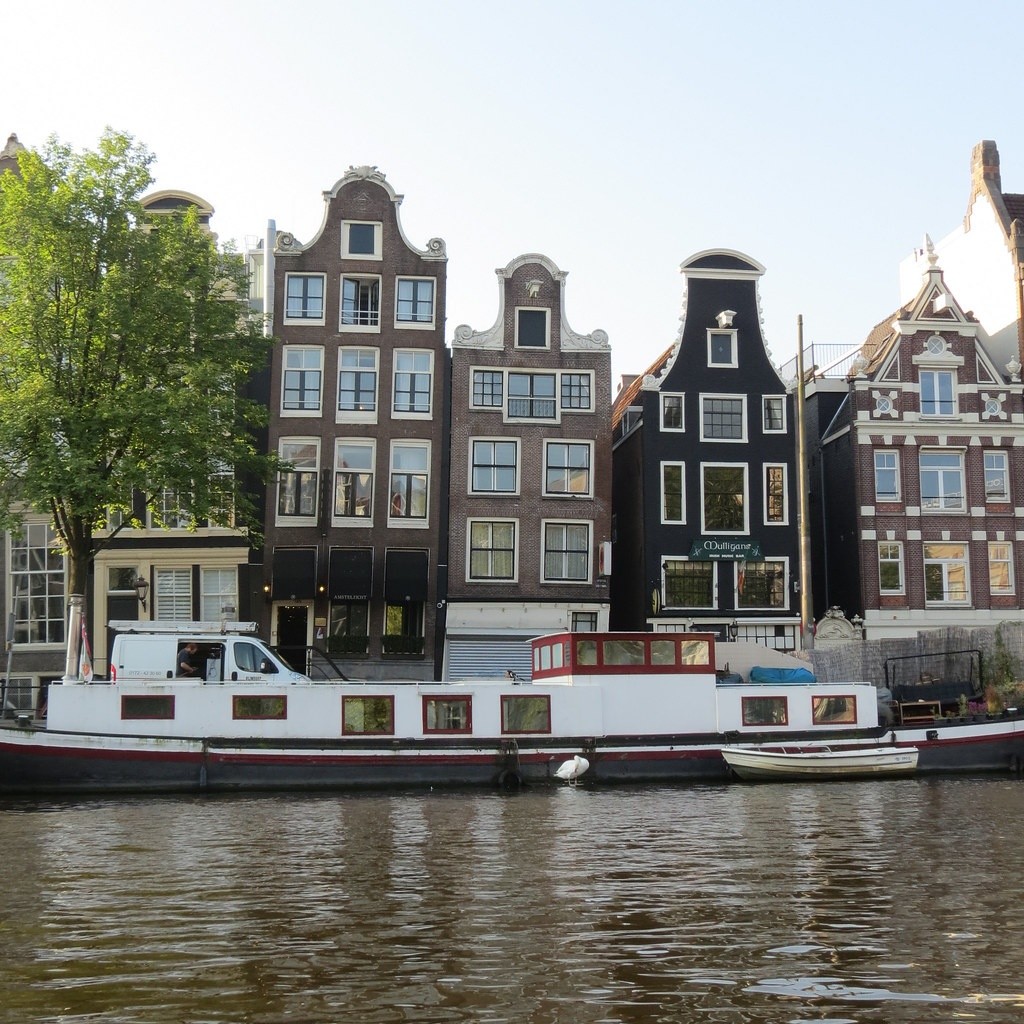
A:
<box><xmin>729</xmin><ymin>622</ymin><xmax>739</xmax><ymax>643</ymax></box>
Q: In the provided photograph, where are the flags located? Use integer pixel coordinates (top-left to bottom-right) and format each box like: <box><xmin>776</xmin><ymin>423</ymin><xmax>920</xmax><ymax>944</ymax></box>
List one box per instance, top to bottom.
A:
<box><xmin>79</xmin><ymin>623</ymin><xmax>94</xmax><ymax>684</ymax></box>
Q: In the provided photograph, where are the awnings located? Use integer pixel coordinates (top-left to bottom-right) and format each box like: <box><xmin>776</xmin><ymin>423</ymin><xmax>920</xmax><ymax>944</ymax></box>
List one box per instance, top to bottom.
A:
<box><xmin>688</xmin><ymin>541</ymin><xmax>765</xmax><ymax>563</ymax></box>
<box><xmin>271</xmin><ymin>549</ymin><xmax>316</xmax><ymax>600</ymax></box>
<box><xmin>329</xmin><ymin>550</ymin><xmax>370</xmax><ymax>603</ymax></box>
<box><xmin>385</xmin><ymin>551</ymin><xmax>427</xmax><ymax>602</ymax></box>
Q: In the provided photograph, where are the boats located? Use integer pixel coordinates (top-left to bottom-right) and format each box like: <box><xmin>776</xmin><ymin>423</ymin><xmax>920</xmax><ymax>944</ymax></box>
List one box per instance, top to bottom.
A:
<box><xmin>0</xmin><ymin>620</ymin><xmax>1024</xmax><ymax>792</ymax></box>
<box><xmin>719</xmin><ymin>742</ymin><xmax>919</xmax><ymax>784</ymax></box>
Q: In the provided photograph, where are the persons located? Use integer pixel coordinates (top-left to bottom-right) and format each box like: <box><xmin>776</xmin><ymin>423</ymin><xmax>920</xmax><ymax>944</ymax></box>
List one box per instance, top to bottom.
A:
<box><xmin>176</xmin><ymin>643</ymin><xmax>198</xmax><ymax>677</ymax></box>
